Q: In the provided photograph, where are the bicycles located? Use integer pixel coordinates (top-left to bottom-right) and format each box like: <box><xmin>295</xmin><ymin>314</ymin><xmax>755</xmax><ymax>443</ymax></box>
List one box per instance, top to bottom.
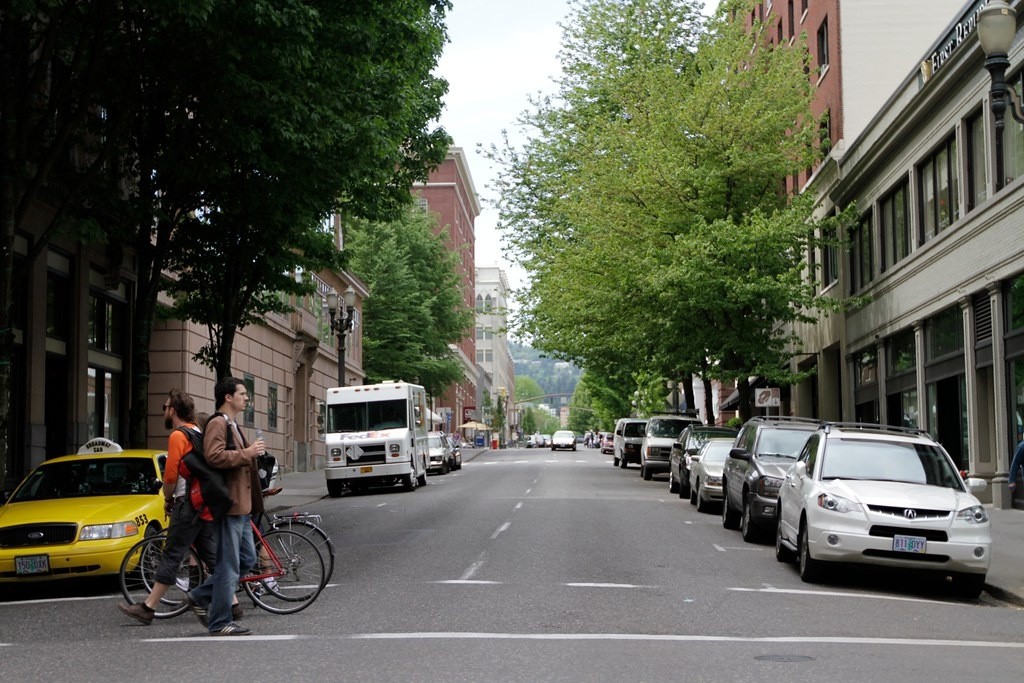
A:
<box><xmin>120</xmin><ymin>486</ymin><xmax>337</xmax><ymax>619</ymax></box>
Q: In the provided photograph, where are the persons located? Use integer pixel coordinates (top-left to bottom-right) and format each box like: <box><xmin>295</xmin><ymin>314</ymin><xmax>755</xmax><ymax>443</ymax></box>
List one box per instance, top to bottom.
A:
<box><xmin>185</xmin><ymin>377</ymin><xmax>265</xmax><ymax>636</ymax></box>
<box><xmin>116</xmin><ymin>386</ymin><xmax>243</xmax><ymax>625</ymax></box>
<box><xmin>387</xmin><ymin>409</ymin><xmax>403</xmax><ymax>425</ymax></box>
<box><xmin>193</xmin><ymin>410</ymin><xmax>209</xmax><ymax>432</ymax></box>
<box><xmin>584</xmin><ymin>429</ymin><xmax>602</xmax><ymax>448</ymax></box>
<box><xmin>452</xmin><ymin>430</ymin><xmax>459</xmax><ymax>444</ymax></box>
<box><xmin>534</xmin><ymin>431</ymin><xmax>539</xmax><ymax>448</ymax></box>
<box><xmin>1008</xmin><ymin>435</ymin><xmax>1024</xmax><ymax>493</ymax></box>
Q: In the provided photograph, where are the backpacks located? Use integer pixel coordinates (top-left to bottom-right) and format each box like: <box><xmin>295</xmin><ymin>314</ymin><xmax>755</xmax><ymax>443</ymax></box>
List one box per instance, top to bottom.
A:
<box><xmin>183</xmin><ymin>411</ymin><xmax>235</xmax><ymax>522</ymax></box>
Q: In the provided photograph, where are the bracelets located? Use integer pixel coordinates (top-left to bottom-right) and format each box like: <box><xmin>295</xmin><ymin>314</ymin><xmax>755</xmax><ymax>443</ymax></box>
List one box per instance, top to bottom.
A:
<box><xmin>163</xmin><ymin>498</ymin><xmax>173</xmax><ymax>503</ymax></box>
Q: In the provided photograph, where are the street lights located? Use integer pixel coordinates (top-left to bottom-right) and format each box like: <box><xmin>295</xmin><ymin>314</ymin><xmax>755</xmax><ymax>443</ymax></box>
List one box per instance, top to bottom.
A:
<box><xmin>325</xmin><ymin>284</ymin><xmax>356</xmax><ymax>387</ymax></box>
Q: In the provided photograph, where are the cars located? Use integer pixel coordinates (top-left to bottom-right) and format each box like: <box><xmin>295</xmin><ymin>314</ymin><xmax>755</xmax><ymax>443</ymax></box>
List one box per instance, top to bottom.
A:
<box><xmin>774</xmin><ymin>421</ymin><xmax>993</xmax><ymax>603</ymax></box>
<box><xmin>427</xmin><ymin>430</ymin><xmax>461</xmax><ymax>476</ymax></box>
<box><xmin>551</xmin><ymin>430</ymin><xmax>577</xmax><ymax>451</ymax></box>
<box><xmin>584</xmin><ymin>431</ymin><xmax>614</xmax><ymax>454</ymax></box>
<box><xmin>525</xmin><ymin>434</ymin><xmax>552</xmax><ymax>448</ymax></box>
<box><xmin>575</xmin><ymin>435</ymin><xmax>584</xmax><ymax>443</ymax></box>
<box><xmin>0</xmin><ymin>438</ymin><xmax>173</xmax><ymax>598</ymax></box>
<box><xmin>689</xmin><ymin>438</ymin><xmax>737</xmax><ymax>514</ymax></box>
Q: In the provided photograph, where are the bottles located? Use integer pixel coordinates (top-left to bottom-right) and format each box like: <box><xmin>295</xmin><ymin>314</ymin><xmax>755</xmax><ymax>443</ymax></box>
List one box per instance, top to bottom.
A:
<box><xmin>255</xmin><ymin>427</ymin><xmax>266</xmax><ymax>455</ymax></box>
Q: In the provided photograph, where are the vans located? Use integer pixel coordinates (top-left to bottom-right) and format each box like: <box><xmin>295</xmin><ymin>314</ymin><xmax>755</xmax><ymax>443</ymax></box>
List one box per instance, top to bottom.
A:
<box><xmin>316</xmin><ymin>379</ymin><xmax>431</xmax><ymax>498</ymax></box>
<box><xmin>638</xmin><ymin>411</ymin><xmax>704</xmax><ymax>481</ymax></box>
<box><xmin>612</xmin><ymin>417</ymin><xmax>648</xmax><ymax>469</ymax></box>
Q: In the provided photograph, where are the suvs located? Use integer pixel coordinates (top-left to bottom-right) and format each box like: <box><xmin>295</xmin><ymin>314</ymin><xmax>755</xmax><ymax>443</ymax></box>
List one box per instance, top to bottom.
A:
<box><xmin>721</xmin><ymin>414</ymin><xmax>825</xmax><ymax>545</ymax></box>
<box><xmin>668</xmin><ymin>424</ymin><xmax>740</xmax><ymax>499</ymax></box>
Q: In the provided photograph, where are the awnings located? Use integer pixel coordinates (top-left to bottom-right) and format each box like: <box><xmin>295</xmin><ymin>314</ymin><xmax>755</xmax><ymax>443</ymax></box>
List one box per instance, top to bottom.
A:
<box><xmin>719</xmin><ymin>377</ymin><xmax>764</xmax><ymax>411</ymax></box>
<box><xmin>680</xmin><ymin>398</ymin><xmax>687</xmax><ymax>409</ymax></box>
<box><xmin>415</xmin><ymin>406</ymin><xmax>442</xmax><ymax>422</ymax></box>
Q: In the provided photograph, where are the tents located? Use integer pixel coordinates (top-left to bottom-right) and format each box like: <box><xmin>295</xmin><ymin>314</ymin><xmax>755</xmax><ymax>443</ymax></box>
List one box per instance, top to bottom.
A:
<box><xmin>457</xmin><ymin>421</ymin><xmax>493</xmax><ymax>448</ymax></box>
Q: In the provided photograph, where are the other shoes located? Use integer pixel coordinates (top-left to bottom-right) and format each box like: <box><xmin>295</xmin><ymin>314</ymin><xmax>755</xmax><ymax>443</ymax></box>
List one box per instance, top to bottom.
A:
<box><xmin>257</xmin><ymin>576</ymin><xmax>279</xmax><ymax>595</ymax></box>
<box><xmin>187</xmin><ymin>589</ymin><xmax>210</xmax><ymax>628</ymax></box>
<box><xmin>117</xmin><ymin>601</ymin><xmax>154</xmax><ymax>625</ymax></box>
<box><xmin>231</xmin><ymin>603</ymin><xmax>243</xmax><ymax>621</ymax></box>
<box><xmin>207</xmin><ymin>623</ymin><xmax>252</xmax><ymax>636</ymax></box>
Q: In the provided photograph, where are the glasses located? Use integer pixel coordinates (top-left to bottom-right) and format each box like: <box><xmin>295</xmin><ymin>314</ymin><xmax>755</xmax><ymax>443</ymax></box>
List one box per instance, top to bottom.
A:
<box><xmin>162</xmin><ymin>404</ymin><xmax>175</xmax><ymax>411</ymax></box>
